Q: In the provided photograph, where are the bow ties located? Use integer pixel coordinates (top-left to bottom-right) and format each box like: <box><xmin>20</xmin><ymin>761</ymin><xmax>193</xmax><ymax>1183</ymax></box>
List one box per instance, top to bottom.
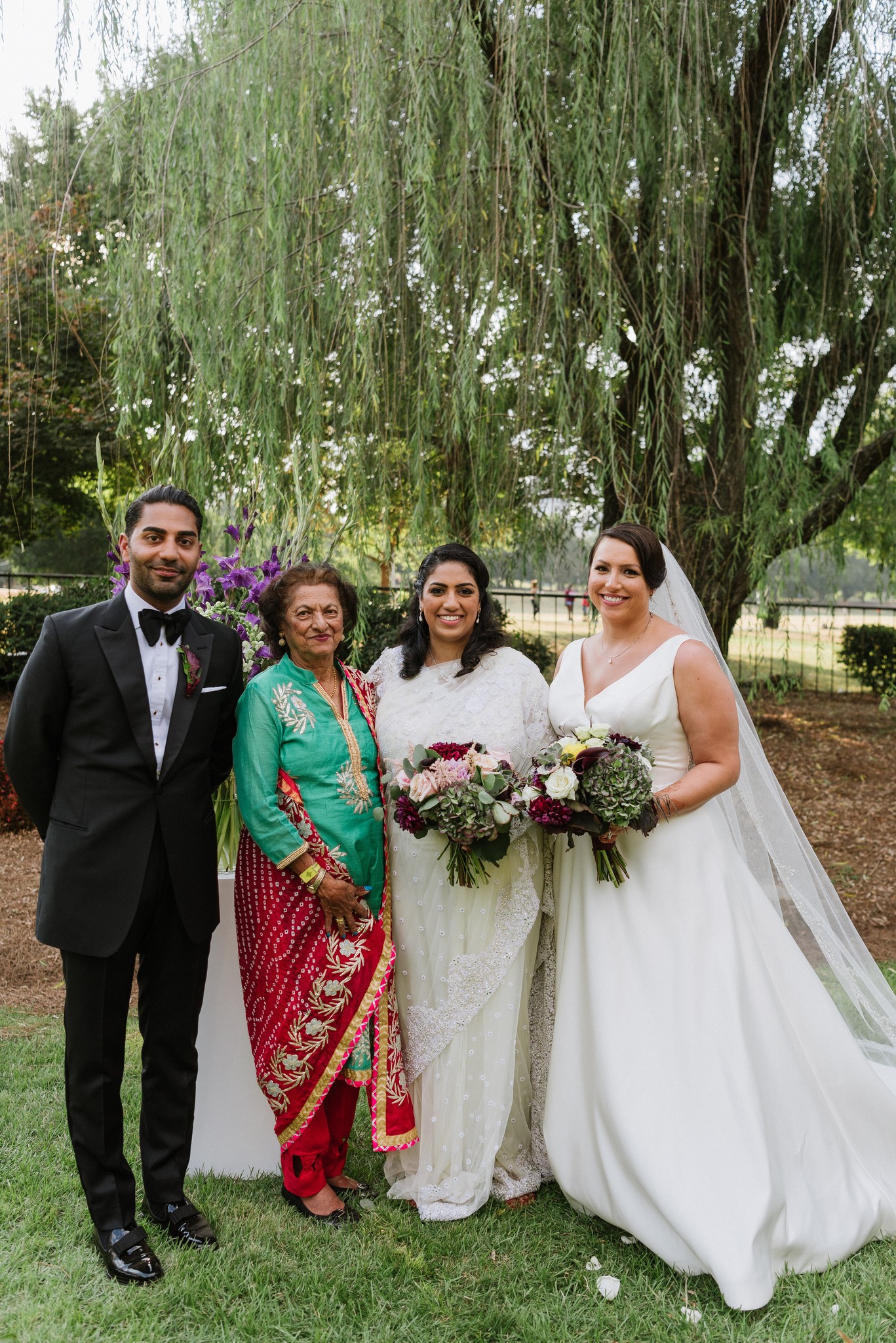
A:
<box><xmin>138</xmin><ymin>609</ymin><xmax>192</xmax><ymax>646</ymax></box>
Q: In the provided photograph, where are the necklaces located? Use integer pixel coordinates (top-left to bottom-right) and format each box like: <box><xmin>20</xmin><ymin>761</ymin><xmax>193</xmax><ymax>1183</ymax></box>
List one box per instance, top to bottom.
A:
<box><xmin>601</xmin><ymin>612</ymin><xmax>653</xmax><ymax>664</ymax></box>
<box><xmin>428</xmin><ymin>645</ymin><xmax>456</xmax><ymax>682</ymax></box>
<box><xmin>329</xmin><ymin>668</ymin><xmax>336</xmax><ymax>698</ymax></box>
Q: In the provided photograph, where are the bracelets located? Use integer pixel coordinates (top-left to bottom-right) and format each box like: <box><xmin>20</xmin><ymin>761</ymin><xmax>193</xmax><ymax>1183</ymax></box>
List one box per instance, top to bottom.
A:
<box><xmin>299</xmin><ymin>862</ymin><xmax>321</xmax><ymax>883</ymax></box>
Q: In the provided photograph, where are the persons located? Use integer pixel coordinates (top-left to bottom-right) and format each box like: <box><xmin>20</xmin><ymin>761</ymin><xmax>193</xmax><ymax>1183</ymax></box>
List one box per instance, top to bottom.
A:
<box><xmin>4</xmin><ymin>487</ymin><xmax>245</xmax><ymax>1286</ymax></box>
<box><xmin>545</xmin><ymin>522</ymin><xmax>896</xmax><ymax>1312</ymax></box>
<box><xmin>363</xmin><ymin>542</ymin><xmax>558</xmax><ymax>1223</ymax></box>
<box><xmin>230</xmin><ymin>559</ymin><xmax>419</xmax><ymax>1227</ymax></box>
<box><xmin>531</xmin><ymin>579</ymin><xmax>540</xmax><ymax>620</ymax></box>
<box><xmin>565</xmin><ymin>584</ymin><xmax>575</xmax><ymax>621</ymax></box>
<box><xmin>582</xmin><ymin>589</ymin><xmax>590</xmax><ymax>622</ymax></box>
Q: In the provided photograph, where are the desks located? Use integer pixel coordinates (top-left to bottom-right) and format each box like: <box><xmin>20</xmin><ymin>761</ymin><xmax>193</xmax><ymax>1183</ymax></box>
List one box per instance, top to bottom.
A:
<box><xmin>185</xmin><ymin>866</ymin><xmax>281</xmax><ymax>1180</ymax></box>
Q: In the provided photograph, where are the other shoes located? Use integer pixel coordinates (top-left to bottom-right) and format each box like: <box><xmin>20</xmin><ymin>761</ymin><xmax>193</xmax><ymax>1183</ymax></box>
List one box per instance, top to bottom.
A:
<box><xmin>507</xmin><ymin>1191</ymin><xmax>534</xmax><ymax>1211</ymax></box>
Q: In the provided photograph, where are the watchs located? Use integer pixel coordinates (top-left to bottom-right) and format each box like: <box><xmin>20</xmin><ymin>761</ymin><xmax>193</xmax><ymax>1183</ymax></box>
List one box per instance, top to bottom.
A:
<box><xmin>306</xmin><ymin>867</ymin><xmax>326</xmax><ymax>894</ymax></box>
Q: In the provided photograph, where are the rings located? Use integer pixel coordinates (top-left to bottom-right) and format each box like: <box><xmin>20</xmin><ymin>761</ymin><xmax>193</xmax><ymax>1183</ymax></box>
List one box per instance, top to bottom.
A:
<box><xmin>607</xmin><ymin>829</ymin><xmax>612</xmax><ymax>840</ymax></box>
<box><xmin>335</xmin><ymin>918</ymin><xmax>345</xmax><ymax>928</ymax></box>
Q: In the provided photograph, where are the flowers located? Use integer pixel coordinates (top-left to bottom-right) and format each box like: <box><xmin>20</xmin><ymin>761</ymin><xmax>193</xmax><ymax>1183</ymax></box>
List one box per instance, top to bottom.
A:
<box><xmin>107</xmin><ymin>491</ymin><xmax>309</xmax><ymax>682</ymax></box>
<box><xmin>176</xmin><ymin>644</ymin><xmax>202</xmax><ymax>696</ymax></box>
<box><xmin>524</xmin><ymin>714</ymin><xmax>657</xmax><ymax>888</ymax></box>
<box><xmin>373</xmin><ymin>739</ymin><xmax>527</xmax><ymax>889</ymax></box>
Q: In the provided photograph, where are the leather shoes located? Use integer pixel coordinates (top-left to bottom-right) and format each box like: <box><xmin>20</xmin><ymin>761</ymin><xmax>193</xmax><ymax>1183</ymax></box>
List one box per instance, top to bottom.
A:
<box><xmin>327</xmin><ymin>1177</ymin><xmax>377</xmax><ymax>1201</ymax></box>
<box><xmin>281</xmin><ymin>1181</ymin><xmax>363</xmax><ymax>1228</ymax></box>
<box><xmin>142</xmin><ymin>1195</ymin><xmax>219</xmax><ymax>1251</ymax></box>
<box><xmin>91</xmin><ymin>1223</ymin><xmax>164</xmax><ymax>1286</ymax></box>
